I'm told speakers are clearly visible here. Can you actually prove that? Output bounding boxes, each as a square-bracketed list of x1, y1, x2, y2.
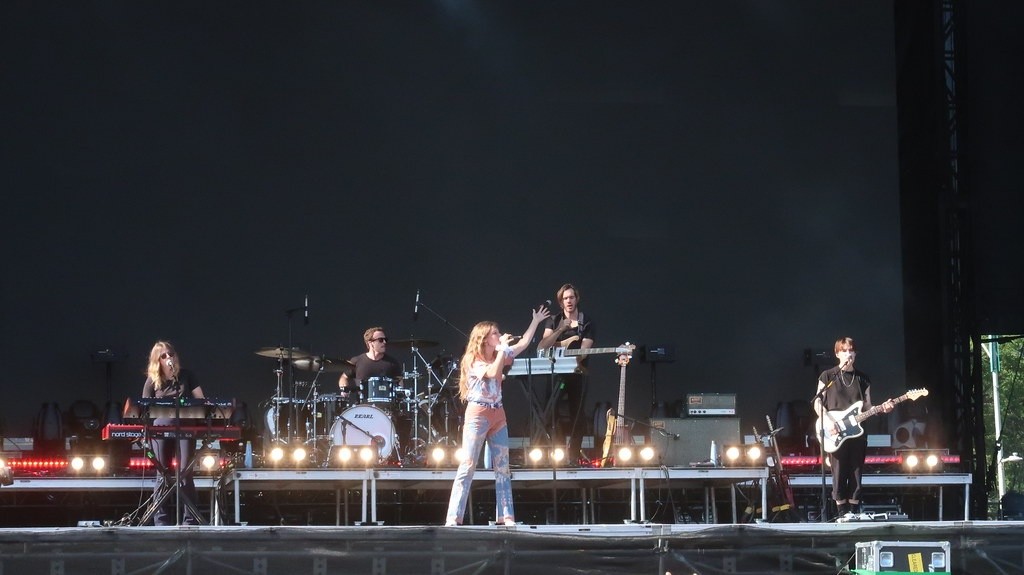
[649, 417, 741, 467]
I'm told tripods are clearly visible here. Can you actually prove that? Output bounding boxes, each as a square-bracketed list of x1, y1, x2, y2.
[296, 363, 330, 467]
[401, 346, 465, 468]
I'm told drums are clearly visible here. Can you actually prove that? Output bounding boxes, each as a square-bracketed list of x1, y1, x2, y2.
[272, 399, 326, 441]
[316, 392, 351, 440]
[359, 376, 394, 409]
[329, 404, 397, 464]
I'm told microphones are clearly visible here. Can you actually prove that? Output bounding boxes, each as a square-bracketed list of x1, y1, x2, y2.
[377, 439, 383, 445]
[304, 295, 309, 324]
[673, 434, 679, 440]
[847, 355, 853, 362]
[413, 290, 419, 321]
[166, 359, 174, 366]
[499, 336, 524, 342]
[541, 300, 552, 311]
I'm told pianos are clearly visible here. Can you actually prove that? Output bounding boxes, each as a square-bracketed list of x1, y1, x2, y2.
[101, 415, 244, 528]
[507, 354, 587, 447]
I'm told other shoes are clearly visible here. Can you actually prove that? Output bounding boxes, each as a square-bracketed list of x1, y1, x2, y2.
[444, 520, 458, 526]
[568, 460, 581, 468]
[844, 511, 871, 521]
[501, 521, 516, 525]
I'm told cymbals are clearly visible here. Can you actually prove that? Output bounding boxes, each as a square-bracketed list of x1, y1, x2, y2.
[290, 355, 357, 373]
[253, 346, 312, 359]
[389, 338, 440, 348]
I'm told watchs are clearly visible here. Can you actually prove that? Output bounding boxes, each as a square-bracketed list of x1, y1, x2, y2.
[340, 387, 349, 392]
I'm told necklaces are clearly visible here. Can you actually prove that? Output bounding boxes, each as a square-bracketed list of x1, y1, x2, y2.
[841, 368, 855, 387]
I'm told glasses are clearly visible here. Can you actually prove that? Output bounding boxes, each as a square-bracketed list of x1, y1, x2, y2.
[370, 337, 388, 342]
[160, 353, 174, 359]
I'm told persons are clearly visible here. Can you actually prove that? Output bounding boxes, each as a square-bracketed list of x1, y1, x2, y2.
[445, 305, 550, 526]
[338, 327, 415, 459]
[537, 284, 595, 467]
[813, 338, 894, 518]
[142, 341, 206, 526]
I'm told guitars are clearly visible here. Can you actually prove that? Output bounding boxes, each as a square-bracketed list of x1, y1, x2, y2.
[751, 414, 795, 521]
[601, 341, 635, 466]
[814, 386, 929, 455]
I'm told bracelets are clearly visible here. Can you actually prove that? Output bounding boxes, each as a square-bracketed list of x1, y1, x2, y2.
[496, 345, 511, 353]
